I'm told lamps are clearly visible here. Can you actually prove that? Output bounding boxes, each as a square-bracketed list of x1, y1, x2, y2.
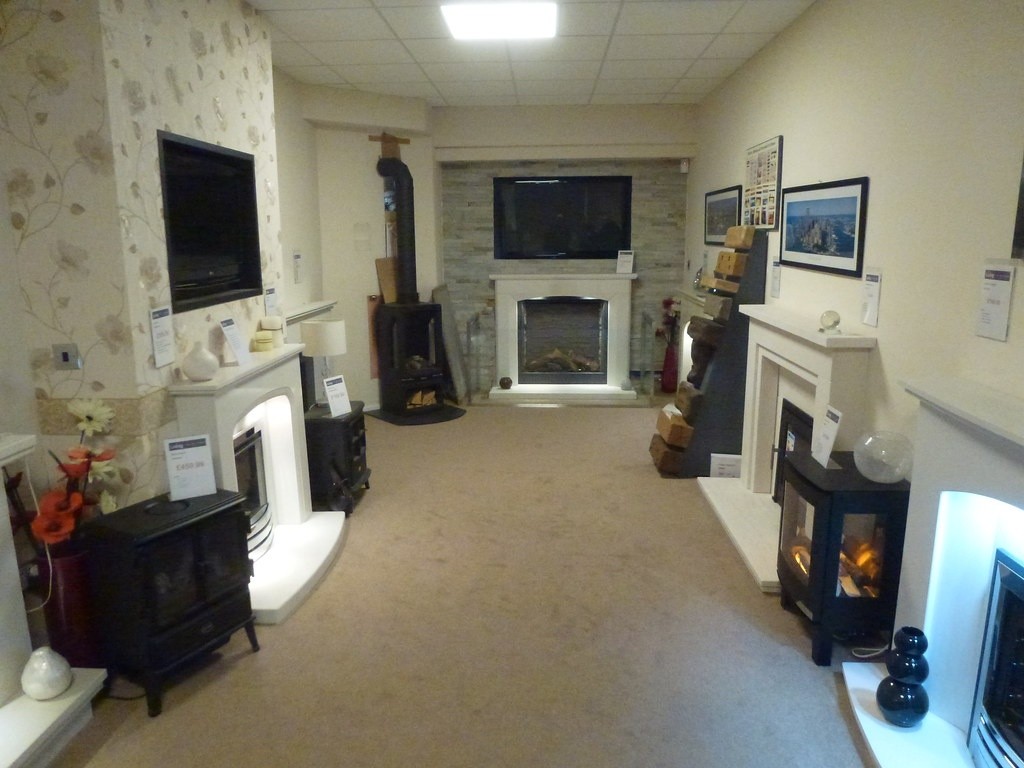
[300, 318, 346, 408]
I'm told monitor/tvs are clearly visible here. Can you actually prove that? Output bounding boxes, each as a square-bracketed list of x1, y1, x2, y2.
[163, 141, 250, 300]
[492, 175, 632, 260]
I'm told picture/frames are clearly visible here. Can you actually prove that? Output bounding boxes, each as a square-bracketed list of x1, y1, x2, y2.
[704, 185, 743, 246]
[779, 176, 869, 278]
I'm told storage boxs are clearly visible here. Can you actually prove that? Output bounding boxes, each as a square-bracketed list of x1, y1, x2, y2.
[649, 225, 756, 473]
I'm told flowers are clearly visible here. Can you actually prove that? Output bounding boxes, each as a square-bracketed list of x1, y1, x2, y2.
[28, 396, 119, 557]
[655, 298, 681, 346]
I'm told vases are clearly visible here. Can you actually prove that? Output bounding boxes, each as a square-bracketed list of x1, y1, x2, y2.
[21, 645, 73, 702]
[182, 341, 219, 382]
[876, 627, 930, 727]
[662, 346, 677, 393]
[38, 542, 116, 698]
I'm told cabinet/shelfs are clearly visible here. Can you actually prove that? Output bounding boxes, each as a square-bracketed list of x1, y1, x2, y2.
[674, 228, 768, 477]
[82, 488, 260, 717]
[304, 401, 372, 518]
